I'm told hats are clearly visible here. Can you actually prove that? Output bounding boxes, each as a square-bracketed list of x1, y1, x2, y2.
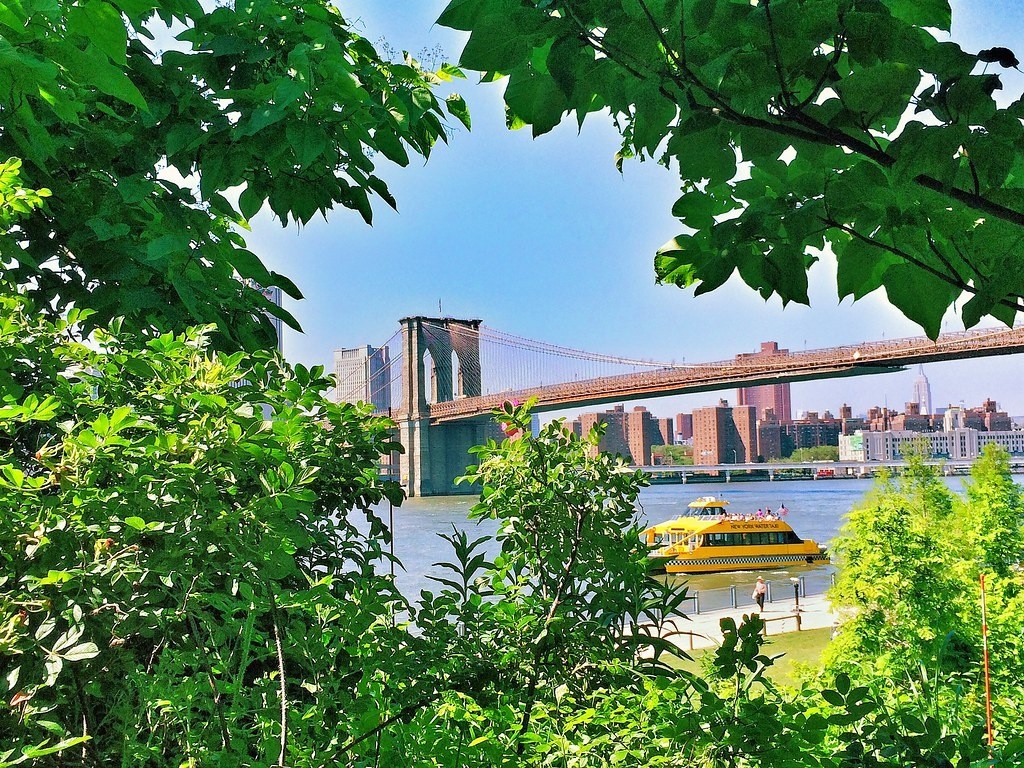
[756, 576, 764, 581]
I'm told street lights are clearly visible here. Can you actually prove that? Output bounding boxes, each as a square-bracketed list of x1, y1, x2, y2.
[733, 450, 737, 464]
[669, 451, 673, 465]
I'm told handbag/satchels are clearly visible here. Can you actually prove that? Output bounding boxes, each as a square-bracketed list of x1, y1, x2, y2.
[752, 589, 759, 600]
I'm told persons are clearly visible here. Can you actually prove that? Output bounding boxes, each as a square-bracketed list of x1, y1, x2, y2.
[717, 512, 745, 521]
[755, 575, 766, 612]
[749, 502, 790, 522]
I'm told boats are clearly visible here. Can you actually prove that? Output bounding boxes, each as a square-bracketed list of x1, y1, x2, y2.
[629, 495, 831, 574]
